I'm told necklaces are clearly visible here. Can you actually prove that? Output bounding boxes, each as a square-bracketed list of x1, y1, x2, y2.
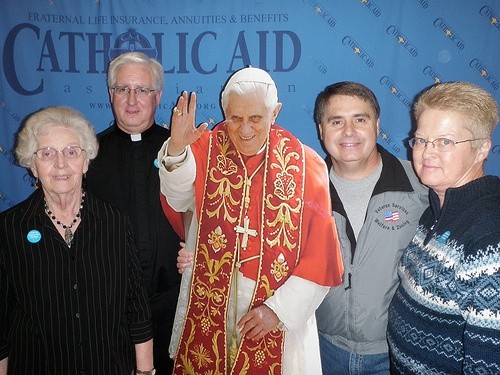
[44, 188, 86, 245]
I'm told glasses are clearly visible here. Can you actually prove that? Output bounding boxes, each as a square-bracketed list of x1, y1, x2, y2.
[112, 86, 155, 96]
[35, 146, 85, 161]
[408, 137, 487, 152]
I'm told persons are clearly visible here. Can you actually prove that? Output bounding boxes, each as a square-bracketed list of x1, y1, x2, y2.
[178, 81, 430, 375]
[386, 81, 500, 375]
[82, 50, 185, 374]
[0, 106, 156, 375]
[155, 66, 345, 374]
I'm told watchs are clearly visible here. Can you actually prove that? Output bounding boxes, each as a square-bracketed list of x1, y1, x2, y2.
[136, 368, 155, 374]
